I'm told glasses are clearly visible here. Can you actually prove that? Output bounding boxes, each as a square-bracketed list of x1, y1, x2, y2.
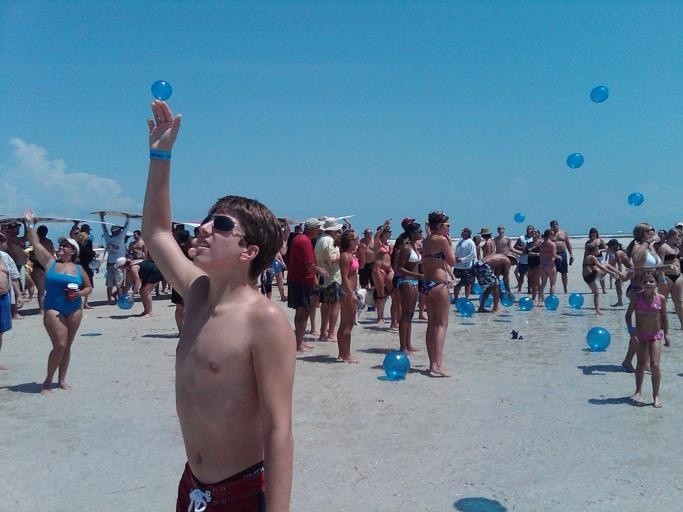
[383, 230, 392, 233]
[60, 244, 67, 247]
[440, 223, 451, 227]
[646, 227, 656, 232]
[415, 230, 424, 233]
[200, 214, 250, 246]
[498, 228, 505, 229]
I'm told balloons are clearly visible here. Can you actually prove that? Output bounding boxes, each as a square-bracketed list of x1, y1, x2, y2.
[586, 328, 611, 351]
[518, 297, 532, 311]
[628, 194, 643, 206]
[479, 294, 494, 308]
[569, 294, 583, 309]
[460, 301, 474, 318]
[456, 297, 467, 314]
[566, 154, 583, 169]
[500, 293, 515, 307]
[545, 295, 559, 311]
[514, 212, 524, 222]
[117, 294, 133, 310]
[473, 284, 483, 295]
[383, 352, 409, 380]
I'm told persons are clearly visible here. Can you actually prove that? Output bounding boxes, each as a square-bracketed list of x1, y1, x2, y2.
[31, 225, 55, 315]
[374, 218, 396, 329]
[172, 230, 193, 336]
[392, 217, 416, 257]
[68, 221, 80, 244]
[396, 223, 428, 354]
[515, 225, 536, 295]
[172, 224, 198, 245]
[629, 270, 671, 408]
[23, 243, 34, 301]
[154, 277, 172, 298]
[422, 213, 456, 376]
[550, 221, 574, 294]
[675, 222, 682, 277]
[112, 257, 163, 318]
[356, 228, 374, 291]
[79, 224, 95, 308]
[473, 235, 482, 261]
[605, 244, 619, 290]
[608, 240, 636, 307]
[583, 243, 624, 314]
[23, 207, 93, 395]
[626, 264, 641, 332]
[657, 228, 683, 330]
[0, 231, 23, 371]
[313, 218, 341, 341]
[476, 253, 517, 313]
[334, 224, 361, 255]
[141, 99, 295, 512]
[337, 230, 365, 363]
[127, 230, 147, 260]
[101, 212, 131, 305]
[493, 225, 523, 255]
[265, 253, 286, 301]
[287, 217, 330, 354]
[478, 228, 495, 257]
[452, 227, 477, 305]
[654, 230, 668, 250]
[622, 223, 662, 371]
[536, 230, 563, 307]
[513, 265, 528, 289]
[6, 221, 26, 319]
[416, 237, 427, 320]
[586, 228, 609, 295]
[527, 229, 543, 303]
[277, 218, 288, 278]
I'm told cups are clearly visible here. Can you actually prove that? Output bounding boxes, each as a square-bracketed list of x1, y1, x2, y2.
[66, 282, 78, 304]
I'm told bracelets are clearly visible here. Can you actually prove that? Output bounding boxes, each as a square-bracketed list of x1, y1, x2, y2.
[148, 151, 171, 161]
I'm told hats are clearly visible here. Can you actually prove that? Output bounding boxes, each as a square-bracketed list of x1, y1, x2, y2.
[113, 256, 126, 269]
[11, 223, 22, 228]
[305, 218, 325, 229]
[58, 235, 80, 257]
[111, 225, 121, 232]
[477, 227, 492, 235]
[319, 217, 343, 231]
[81, 224, 92, 231]
[36, 225, 48, 234]
[400, 217, 416, 229]
[675, 222, 683, 228]
